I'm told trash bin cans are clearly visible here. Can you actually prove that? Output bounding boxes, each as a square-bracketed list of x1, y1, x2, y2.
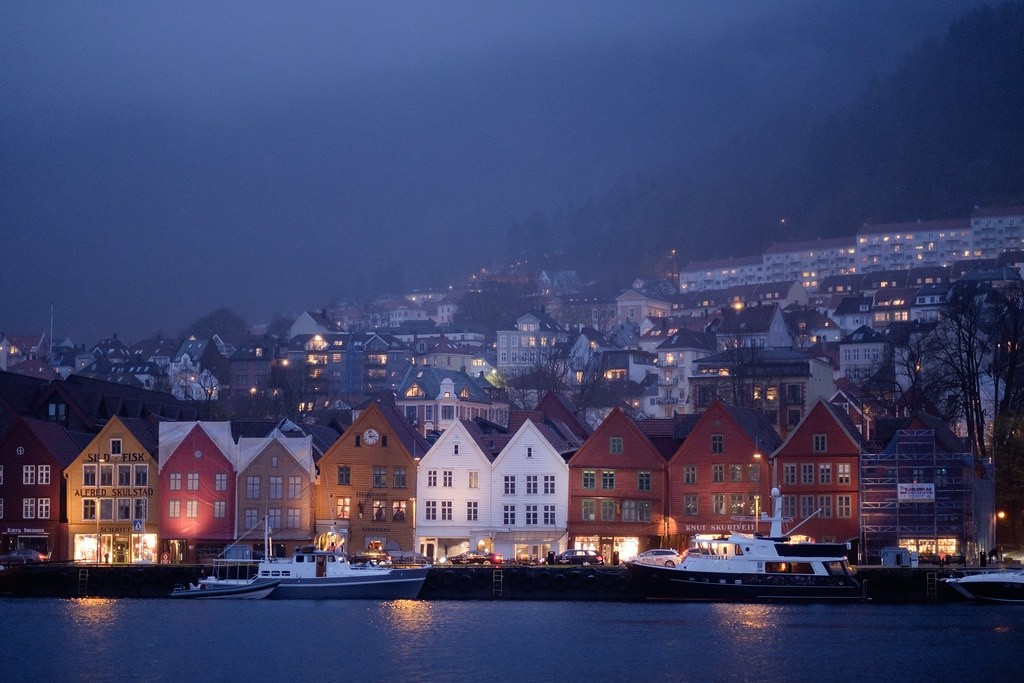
[979, 552, 986, 567]
[548, 550, 555, 565]
[613, 551, 619, 565]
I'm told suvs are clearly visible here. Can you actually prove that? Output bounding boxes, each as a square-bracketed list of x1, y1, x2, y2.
[632, 549, 683, 568]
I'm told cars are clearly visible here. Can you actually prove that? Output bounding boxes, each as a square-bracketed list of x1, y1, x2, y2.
[444, 549, 499, 566]
[0, 548, 44, 564]
[554, 549, 603, 566]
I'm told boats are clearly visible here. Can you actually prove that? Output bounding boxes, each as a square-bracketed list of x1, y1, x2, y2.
[168, 558, 282, 599]
[937, 567, 1024, 603]
[621, 509, 873, 604]
[257, 515, 433, 600]
